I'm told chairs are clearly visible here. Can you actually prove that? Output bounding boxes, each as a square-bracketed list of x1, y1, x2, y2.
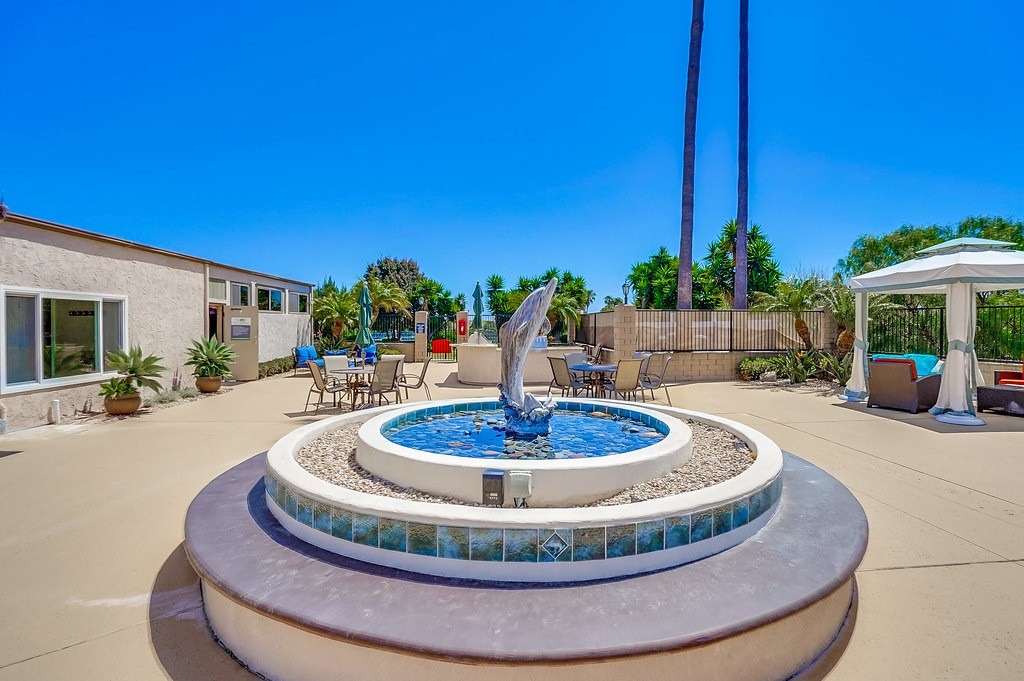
[547, 343, 673, 407]
[291, 343, 432, 415]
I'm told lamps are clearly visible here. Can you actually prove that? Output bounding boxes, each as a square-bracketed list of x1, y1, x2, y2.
[507, 470, 534, 508]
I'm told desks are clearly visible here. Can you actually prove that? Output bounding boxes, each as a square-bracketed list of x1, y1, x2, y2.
[330, 367, 376, 410]
[572, 364, 617, 398]
[347, 357, 367, 367]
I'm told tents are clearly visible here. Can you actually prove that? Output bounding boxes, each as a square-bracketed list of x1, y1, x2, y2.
[844, 238, 1024, 415]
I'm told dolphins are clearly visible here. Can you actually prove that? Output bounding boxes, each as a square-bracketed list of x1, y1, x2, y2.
[498, 277, 559, 414]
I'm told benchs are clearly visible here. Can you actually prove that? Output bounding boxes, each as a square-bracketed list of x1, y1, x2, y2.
[977, 371, 1024, 414]
[871, 353, 945, 377]
[865, 358, 942, 413]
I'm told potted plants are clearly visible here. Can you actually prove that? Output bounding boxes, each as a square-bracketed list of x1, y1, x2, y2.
[99, 344, 164, 414]
[185, 332, 240, 393]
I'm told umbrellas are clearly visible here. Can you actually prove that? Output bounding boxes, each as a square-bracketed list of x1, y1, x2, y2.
[355, 281, 375, 369]
[471, 281, 484, 346]
